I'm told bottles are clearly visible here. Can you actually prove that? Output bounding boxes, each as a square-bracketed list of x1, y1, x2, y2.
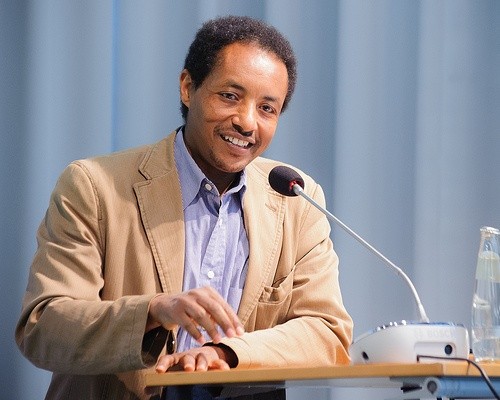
[471, 225, 499, 363]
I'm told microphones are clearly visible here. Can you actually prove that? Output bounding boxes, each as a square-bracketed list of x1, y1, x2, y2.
[269, 165, 469, 363]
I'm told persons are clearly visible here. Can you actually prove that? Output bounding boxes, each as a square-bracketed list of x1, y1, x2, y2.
[13, 13, 354, 400]
